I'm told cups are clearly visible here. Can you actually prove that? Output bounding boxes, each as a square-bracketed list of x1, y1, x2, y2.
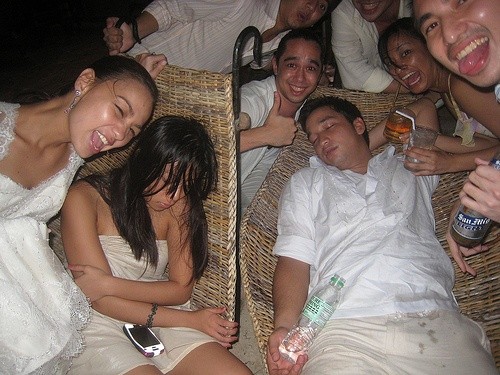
[384, 105, 415, 142]
[404, 125, 438, 172]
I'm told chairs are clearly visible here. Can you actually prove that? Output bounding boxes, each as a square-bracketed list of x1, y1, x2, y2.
[237, 85, 500, 372]
[52, 25, 262, 348]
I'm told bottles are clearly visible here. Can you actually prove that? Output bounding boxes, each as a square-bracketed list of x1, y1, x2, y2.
[450, 149, 500, 246]
[280, 274, 344, 362]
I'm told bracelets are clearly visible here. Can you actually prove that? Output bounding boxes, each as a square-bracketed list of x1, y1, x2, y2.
[131, 18, 142, 44]
[145, 303, 158, 329]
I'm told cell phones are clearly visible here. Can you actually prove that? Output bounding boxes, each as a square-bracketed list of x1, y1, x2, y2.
[124, 323, 166, 357]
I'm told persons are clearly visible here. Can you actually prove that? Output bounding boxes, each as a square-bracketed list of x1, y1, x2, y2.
[102, 0, 342, 74]
[266, 94, 500, 375]
[378, 0, 500, 224]
[60, 115, 254, 375]
[0, 55, 159, 375]
[331, 0, 415, 94]
[240, 29, 326, 153]
